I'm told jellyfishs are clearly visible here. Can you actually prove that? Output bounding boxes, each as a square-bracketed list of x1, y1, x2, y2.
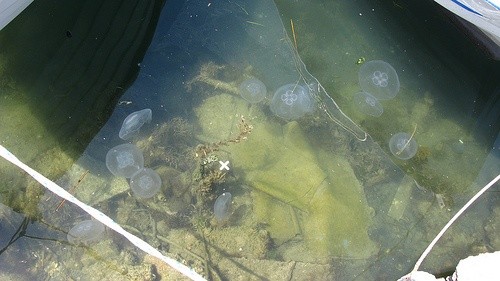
[351, 92, 385, 118]
[66, 218, 105, 246]
[358, 60, 399, 100]
[105, 143, 145, 179]
[239, 77, 267, 105]
[118, 109, 152, 141]
[130, 168, 161, 198]
[389, 131, 418, 160]
[269, 83, 313, 121]
[213, 191, 234, 226]
